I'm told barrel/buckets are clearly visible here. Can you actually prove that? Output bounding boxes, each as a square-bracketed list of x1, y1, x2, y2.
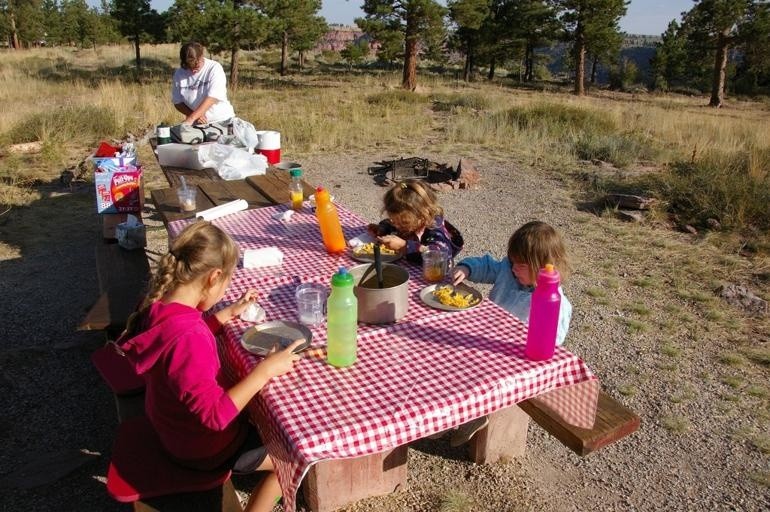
[254, 130, 281, 164]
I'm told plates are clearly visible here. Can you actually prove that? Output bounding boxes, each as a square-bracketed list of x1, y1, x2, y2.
[349, 232, 404, 263]
[420, 281, 483, 312]
[242, 320, 311, 355]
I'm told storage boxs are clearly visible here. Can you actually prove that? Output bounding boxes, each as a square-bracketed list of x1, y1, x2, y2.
[94, 167, 144, 215]
[91, 148, 137, 172]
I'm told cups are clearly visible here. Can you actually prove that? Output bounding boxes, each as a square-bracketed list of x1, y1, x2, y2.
[420, 249, 450, 282]
[295, 285, 327, 327]
[179, 190, 198, 212]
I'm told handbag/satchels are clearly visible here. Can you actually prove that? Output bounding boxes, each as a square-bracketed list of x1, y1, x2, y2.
[170, 122, 237, 144]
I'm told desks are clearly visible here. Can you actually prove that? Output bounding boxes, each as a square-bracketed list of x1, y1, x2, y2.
[149, 138, 284, 188]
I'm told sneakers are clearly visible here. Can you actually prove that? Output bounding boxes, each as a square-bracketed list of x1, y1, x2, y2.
[451, 415, 490, 449]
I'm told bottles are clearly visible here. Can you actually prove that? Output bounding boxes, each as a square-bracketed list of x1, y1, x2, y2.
[315, 186, 347, 253]
[289, 170, 304, 211]
[328, 268, 361, 370]
[157, 122, 171, 145]
[524, 264, 561, 362]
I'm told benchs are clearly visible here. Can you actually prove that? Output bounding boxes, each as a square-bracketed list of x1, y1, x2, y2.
[518, 378, 640, 458]
[94, 243, 245, 512]
[102, 214, 146, 241]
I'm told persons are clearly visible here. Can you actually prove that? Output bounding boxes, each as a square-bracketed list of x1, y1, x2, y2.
[171, 42, 236, 126]
[427, 220, 573, 448]
[366, 179, 464, 269]
[113, 220, 307, 512]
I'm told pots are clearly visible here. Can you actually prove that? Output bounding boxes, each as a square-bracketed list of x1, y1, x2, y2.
[346, 263, 410, 322]
